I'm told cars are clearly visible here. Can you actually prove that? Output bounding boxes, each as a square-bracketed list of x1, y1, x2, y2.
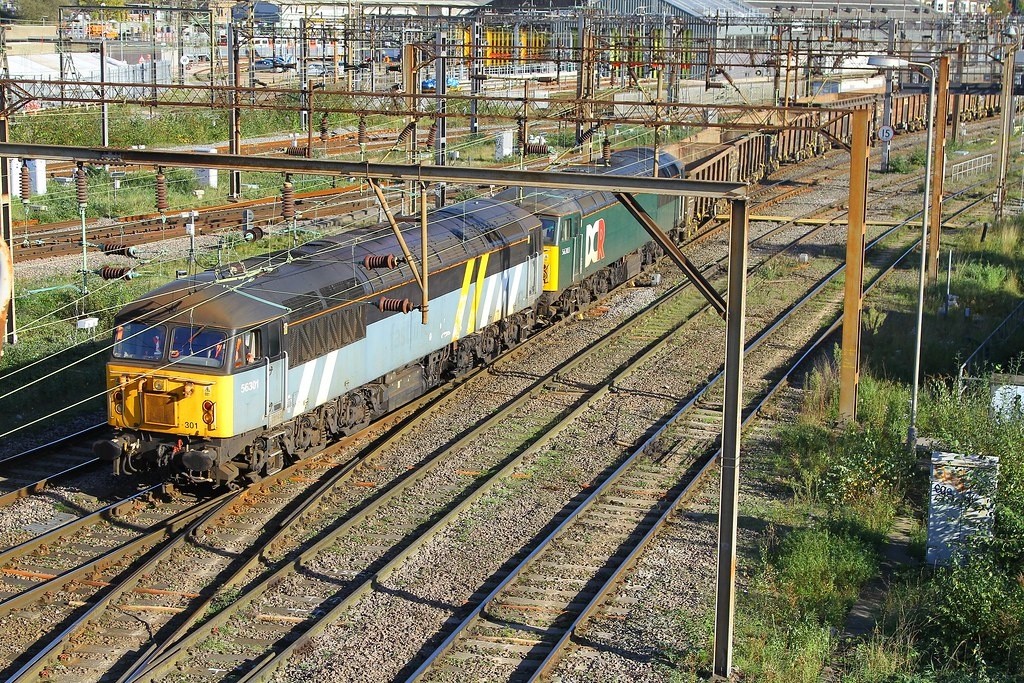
[250, 56, 371, 77]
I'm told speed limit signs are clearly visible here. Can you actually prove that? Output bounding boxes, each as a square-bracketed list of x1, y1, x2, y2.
[877, 125, 894, 142]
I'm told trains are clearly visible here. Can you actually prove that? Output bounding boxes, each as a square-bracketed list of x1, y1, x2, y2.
[93, 81, 1024, 494]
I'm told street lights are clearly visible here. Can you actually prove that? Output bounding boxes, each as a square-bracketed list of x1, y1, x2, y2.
[100, 2, 106, 37]
[867, 57, 936, 467]
[995, 26, 1022, 221]
[109, 20, 123, 61]
[401, 27, 424, 102]
